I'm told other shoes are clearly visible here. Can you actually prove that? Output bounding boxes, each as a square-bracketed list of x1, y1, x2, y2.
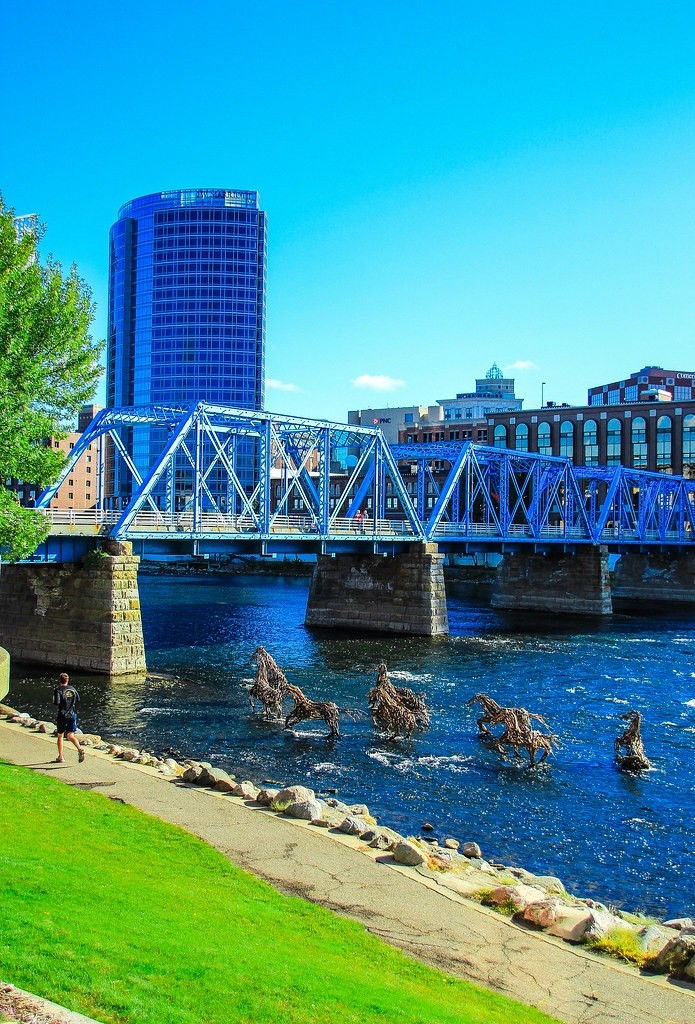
[56, 757, 65, 762]
[78, 748, 85, 763]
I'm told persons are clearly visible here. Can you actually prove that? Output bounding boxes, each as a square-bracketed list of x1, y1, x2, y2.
[26, 496, 36, 508]
[53, 673, 85, 763]
[354, 509, 368, 534]
